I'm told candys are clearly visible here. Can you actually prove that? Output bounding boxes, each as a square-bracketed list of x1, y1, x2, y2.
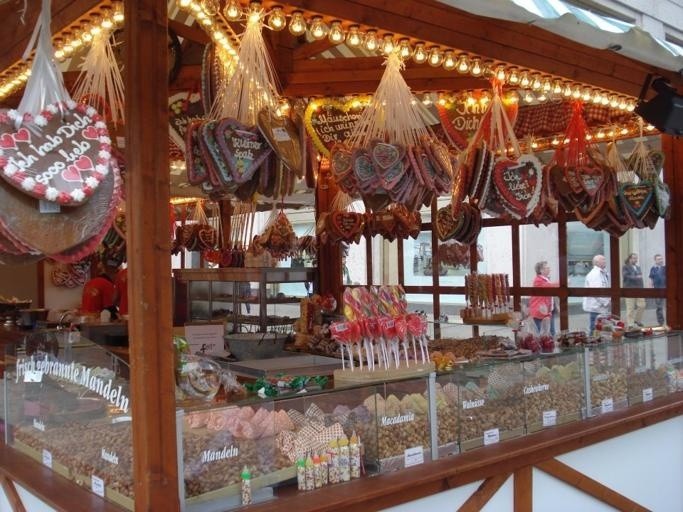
[465, 272, 510, 320]
[321, 284, 431, 371]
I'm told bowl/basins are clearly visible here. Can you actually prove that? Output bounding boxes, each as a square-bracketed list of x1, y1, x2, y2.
[224, 333, 289, 360]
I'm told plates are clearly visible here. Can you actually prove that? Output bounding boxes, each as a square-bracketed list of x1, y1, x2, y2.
[540, 351, 560, 358]
[454, 358, 469, 364]
[474, 349, 532, 360]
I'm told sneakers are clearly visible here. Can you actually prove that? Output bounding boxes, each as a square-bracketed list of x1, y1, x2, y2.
[634, 320, 644, 326]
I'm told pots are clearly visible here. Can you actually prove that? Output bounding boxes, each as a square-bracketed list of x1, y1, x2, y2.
[17, 309, 48, 328]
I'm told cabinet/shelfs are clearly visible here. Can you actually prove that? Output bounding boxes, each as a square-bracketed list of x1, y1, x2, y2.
[172, 268, 318, 333]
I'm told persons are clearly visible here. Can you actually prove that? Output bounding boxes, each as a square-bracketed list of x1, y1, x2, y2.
[528, 261, 559, 336]
[621, 253, 646, 329]
[78, 272, 118, 320]
[582, 254, 612, 336]
[648, 254, 667, 327]
[113, 268, 127, 315]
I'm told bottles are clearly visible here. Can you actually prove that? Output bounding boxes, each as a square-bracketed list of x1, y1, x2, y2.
[241, 465, 253, 507]
[298, 431, 362, 492]
